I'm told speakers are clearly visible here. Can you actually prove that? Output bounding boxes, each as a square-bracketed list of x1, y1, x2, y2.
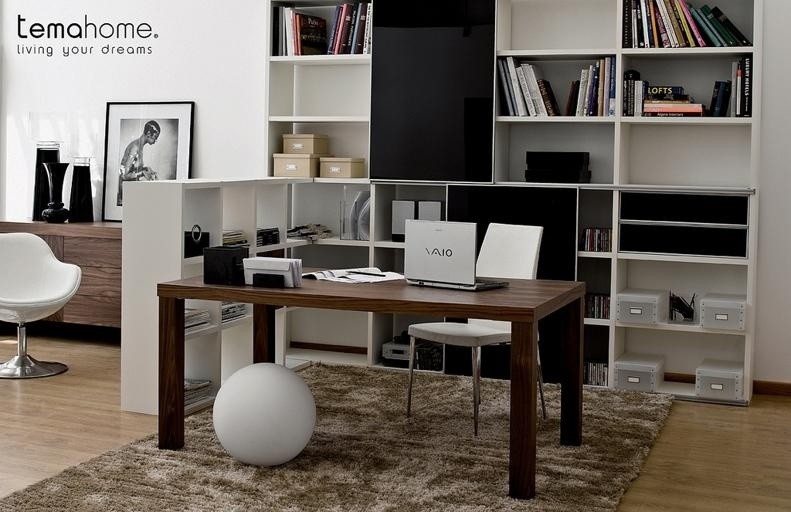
[391, 199, 416, 241]
[418, 199, 442, 221]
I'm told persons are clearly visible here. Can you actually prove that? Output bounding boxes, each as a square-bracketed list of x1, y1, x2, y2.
[116, 118, 162, 207]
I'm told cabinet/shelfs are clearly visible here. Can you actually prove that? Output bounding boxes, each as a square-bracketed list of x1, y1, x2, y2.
[3, 216, 120, 338]
[490, 0, 763, 185]
[289, 181, 761, 409]
[123, 171, 311, 422]
[263, 1, 378, 179]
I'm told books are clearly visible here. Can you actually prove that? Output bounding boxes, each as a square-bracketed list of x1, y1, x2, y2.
[273, 1, 374, 56]
[496, 57, 616, 117]
[582, 224, 613, 387]
[622, 0, 753, 118]
[302, 265, 408, 284]
[184, 298, 249, 408]
[222, 222, 282, 249]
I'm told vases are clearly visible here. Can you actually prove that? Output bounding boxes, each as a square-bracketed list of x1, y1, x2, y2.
[41, 162, 69, 224]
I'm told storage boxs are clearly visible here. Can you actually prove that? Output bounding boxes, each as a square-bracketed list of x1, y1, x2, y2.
[278, 131, 328, 156]
[317, 157, 366, 179]
[271, 153, 320, 179]
[692, 361, 740, 398]
[701, 291, 746, 332]
[611, 351, 665, 393]
[616, 285, 669, 324]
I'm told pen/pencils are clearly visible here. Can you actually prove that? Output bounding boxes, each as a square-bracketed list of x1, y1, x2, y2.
[348, 271, 385, 277]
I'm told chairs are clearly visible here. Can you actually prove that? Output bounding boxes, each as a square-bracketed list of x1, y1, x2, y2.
[0, 231, 78, 381]
[405, 220, 549, 440]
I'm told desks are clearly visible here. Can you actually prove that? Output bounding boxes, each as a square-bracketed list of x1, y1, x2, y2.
[151, 267, 585, 503]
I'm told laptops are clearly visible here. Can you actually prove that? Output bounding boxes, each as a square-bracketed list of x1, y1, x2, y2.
[401, 219, 509, 292]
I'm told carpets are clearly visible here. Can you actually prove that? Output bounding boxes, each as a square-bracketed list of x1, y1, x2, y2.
[1, 355, 671, 512]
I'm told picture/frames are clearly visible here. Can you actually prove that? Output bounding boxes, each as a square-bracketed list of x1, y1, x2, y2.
[102, 96, 194, 224]
